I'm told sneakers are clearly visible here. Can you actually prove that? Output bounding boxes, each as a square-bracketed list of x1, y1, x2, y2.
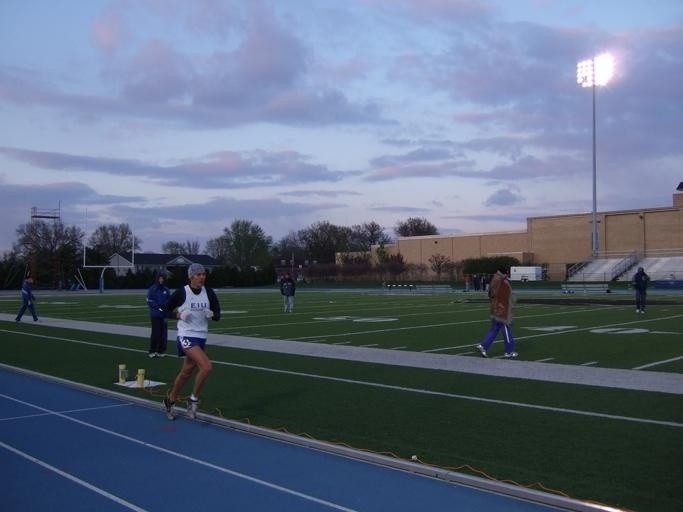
[187, 396, 201, 419]
[504, 352, 520, 359]
[148, 353, 156, 358]
[476, 345, 490, 360]
[158, 352, 169, 358]
[163, 391, 178, 420]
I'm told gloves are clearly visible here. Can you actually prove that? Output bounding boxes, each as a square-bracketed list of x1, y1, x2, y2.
[179, 308, 193, 320]
[204, 307, 214, 319]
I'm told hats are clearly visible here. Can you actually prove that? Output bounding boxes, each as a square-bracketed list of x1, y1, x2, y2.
[187, 262, 207, 278]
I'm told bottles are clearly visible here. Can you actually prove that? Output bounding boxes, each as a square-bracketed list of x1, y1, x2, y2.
[136, 369, 146, 388]
[119, 364, 127, 384]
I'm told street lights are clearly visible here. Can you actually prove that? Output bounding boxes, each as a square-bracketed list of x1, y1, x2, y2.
[576, 52, 617, 255]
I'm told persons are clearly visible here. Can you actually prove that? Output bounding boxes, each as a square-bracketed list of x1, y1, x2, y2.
[144, 273, 169, 358]
[631, 266, 650, 313]
[463, 272, 487, 290]
[162, 263, 221, 422]
[14, 275, 38, 322]
[474, 266, 518, 358]
[279, 271, 294, 312]
